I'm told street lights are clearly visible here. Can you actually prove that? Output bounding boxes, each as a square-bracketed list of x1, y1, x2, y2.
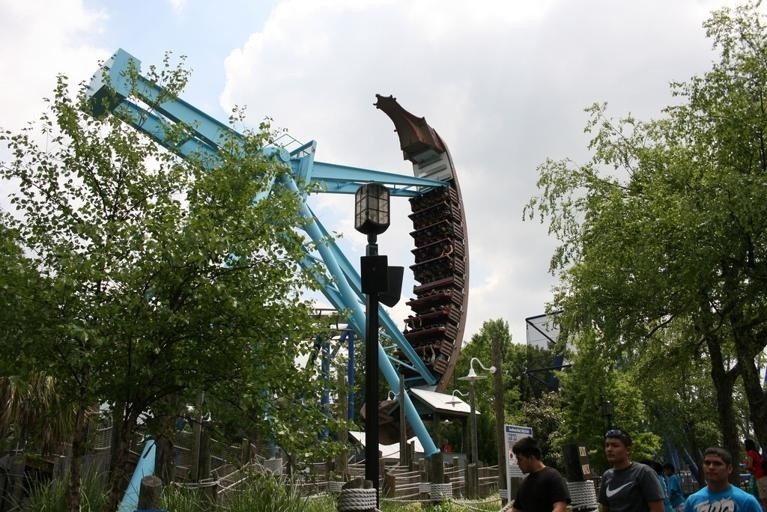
[355, 184, 390, 507]
[601, 396, 614, 426]
[448, 340, 508, 508]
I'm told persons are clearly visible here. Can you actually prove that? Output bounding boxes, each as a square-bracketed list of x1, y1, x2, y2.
[175, 411, 189, 432]
[440, 437, 452, 453]
[506, 436, 571, 512]
[597, 428, 767, 512]
[397, 188, 457, 368]
